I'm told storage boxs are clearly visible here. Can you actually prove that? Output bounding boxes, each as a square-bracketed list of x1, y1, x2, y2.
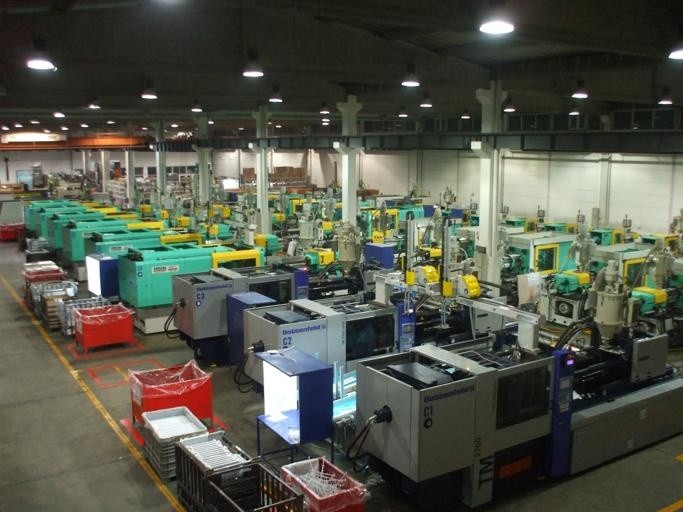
[21, 260, 138, 353]
[127, 359, 365, 511]
